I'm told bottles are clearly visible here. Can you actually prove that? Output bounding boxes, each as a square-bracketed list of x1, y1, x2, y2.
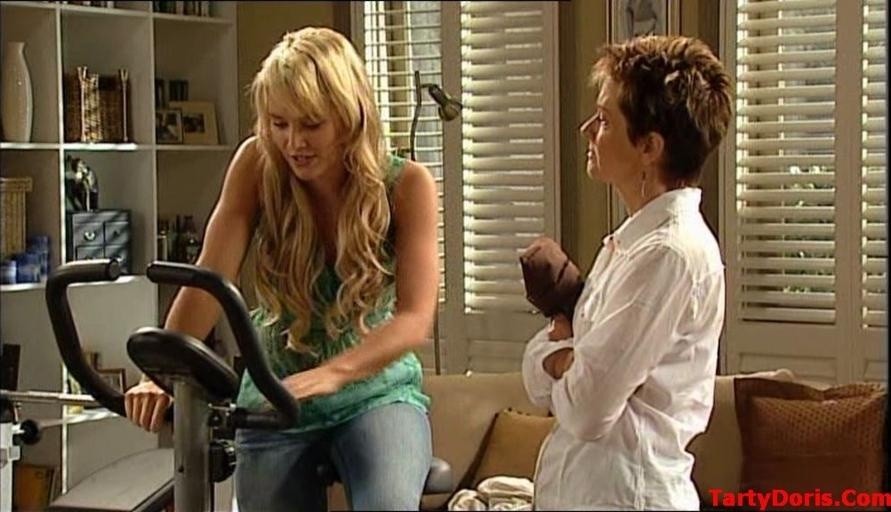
[172, 215, 203, 264]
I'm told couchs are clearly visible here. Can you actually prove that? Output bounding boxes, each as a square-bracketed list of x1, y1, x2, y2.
[416, 360, 887, 510]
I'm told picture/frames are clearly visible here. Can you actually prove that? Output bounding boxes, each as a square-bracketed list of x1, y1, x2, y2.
[157, 99, 223, 147]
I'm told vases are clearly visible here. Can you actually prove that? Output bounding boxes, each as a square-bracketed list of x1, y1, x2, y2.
[2, 39, 37, 142]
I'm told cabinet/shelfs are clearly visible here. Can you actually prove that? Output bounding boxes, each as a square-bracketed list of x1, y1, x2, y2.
[0, 2, 241, 506]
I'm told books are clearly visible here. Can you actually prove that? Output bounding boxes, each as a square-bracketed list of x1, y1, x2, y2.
[29, 0, 216, 17]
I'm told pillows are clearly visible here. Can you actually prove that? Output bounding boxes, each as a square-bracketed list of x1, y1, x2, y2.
[426, 369, 553, 489]
[689, 365, 889, 510]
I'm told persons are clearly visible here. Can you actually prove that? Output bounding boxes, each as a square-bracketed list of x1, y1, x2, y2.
[520, 35, 732, 511]
[126, 26, 439, 512]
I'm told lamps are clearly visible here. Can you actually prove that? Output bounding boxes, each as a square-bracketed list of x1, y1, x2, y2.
[408, 68, 466, 377]
